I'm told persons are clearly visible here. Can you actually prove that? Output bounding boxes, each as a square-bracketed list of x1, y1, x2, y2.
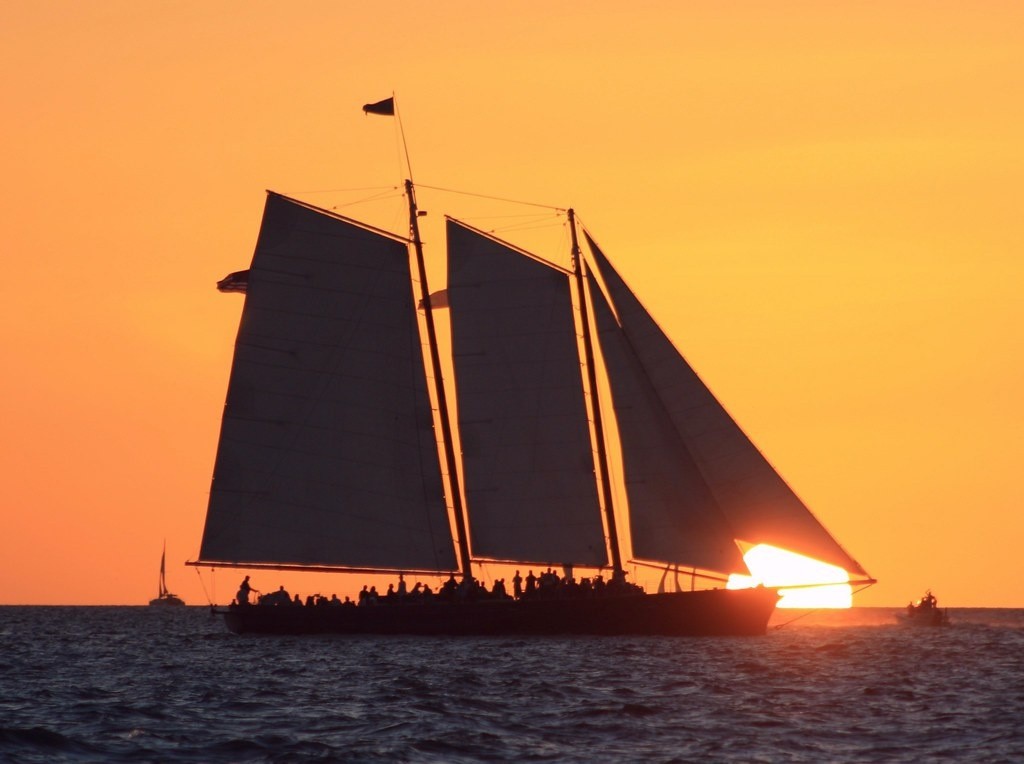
[907, 589, 936, 617]
[236, 576, 259, 606]
[278, 567, 613, 607]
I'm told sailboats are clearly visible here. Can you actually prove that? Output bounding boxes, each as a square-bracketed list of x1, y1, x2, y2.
[181, 88, 878, 637]
[148, 538, 187, 606]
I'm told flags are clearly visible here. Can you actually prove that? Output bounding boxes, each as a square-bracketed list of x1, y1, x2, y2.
[362, 97, 394, 116]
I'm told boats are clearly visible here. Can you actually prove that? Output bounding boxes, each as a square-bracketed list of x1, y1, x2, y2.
[894, 590, 953, 628]
